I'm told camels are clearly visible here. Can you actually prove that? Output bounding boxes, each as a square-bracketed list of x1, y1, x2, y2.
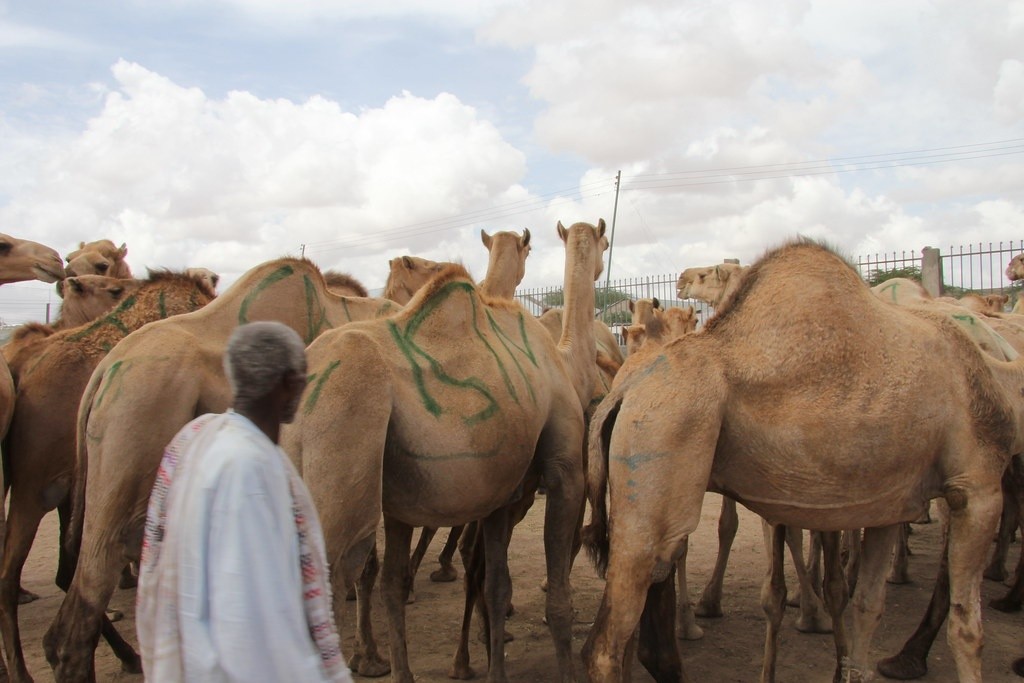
[0, 215, 1023, 682]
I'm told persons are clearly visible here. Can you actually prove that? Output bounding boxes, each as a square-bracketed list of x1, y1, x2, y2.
[136, 321, 355, 683]
